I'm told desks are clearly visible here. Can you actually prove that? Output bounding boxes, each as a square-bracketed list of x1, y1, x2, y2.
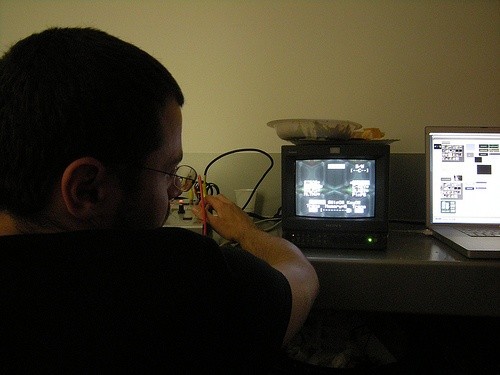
[161, 217, 500, 317]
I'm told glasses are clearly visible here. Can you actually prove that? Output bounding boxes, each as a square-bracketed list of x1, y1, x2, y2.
[56, 162, 197, 192]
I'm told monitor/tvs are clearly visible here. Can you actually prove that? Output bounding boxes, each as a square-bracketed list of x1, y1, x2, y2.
[281, 144, 389, 252]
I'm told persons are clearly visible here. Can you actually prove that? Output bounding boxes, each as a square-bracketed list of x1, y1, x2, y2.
[0, 27, 318, 375]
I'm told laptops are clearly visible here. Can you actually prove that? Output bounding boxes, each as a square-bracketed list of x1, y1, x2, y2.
[424, 126, 500, 259]
